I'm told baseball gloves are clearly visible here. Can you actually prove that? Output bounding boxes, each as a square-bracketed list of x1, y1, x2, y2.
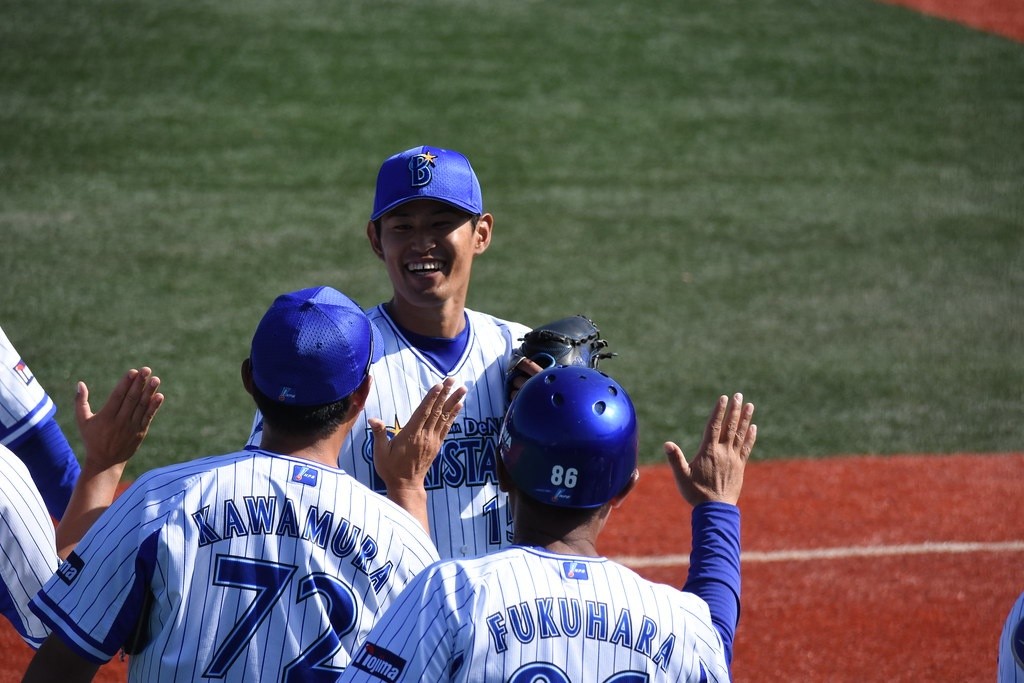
[504, 316, 618, 414]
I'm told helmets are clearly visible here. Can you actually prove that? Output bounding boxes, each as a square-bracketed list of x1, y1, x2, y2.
[499, 365, 638, 511]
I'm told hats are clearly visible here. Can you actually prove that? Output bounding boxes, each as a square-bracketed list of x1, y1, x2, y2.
[370, 144, 483, 224]
[250, 285, 385, 408]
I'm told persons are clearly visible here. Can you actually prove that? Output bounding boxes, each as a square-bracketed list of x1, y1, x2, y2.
[247, 146, 547, 561]
[18, 285, 443, 683]
[0, 321, 166, 648]
[337, 364, 759, 683]
